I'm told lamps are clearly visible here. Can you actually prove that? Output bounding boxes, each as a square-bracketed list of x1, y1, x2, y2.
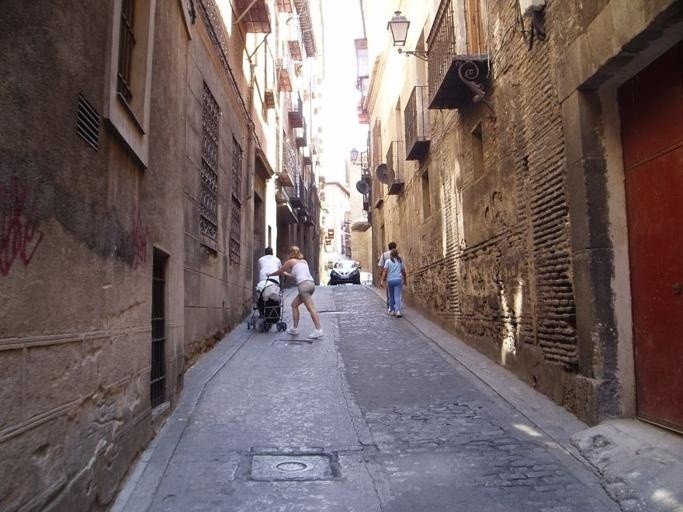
[350, 149, 369, 167]
[387, 11, 428, 60]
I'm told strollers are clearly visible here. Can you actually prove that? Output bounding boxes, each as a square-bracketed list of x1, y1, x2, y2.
[247, 274, 287, 332]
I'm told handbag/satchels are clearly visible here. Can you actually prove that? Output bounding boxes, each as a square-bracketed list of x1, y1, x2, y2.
[379, 266, 386, 278]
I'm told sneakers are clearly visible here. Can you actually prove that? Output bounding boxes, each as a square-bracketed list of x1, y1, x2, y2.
[308, 328, 322, 338]
[387, 306, 402, 317]
[287, 327, 297, 334]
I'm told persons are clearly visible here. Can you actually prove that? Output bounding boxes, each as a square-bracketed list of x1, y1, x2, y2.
[377, 242, 402, 309]
[379, 250, 414, 318]
[256, 246, 284, 288]
[267, 247, 326, 339]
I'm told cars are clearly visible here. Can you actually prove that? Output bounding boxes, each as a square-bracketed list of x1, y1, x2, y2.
[330, 260, 361, 284]
